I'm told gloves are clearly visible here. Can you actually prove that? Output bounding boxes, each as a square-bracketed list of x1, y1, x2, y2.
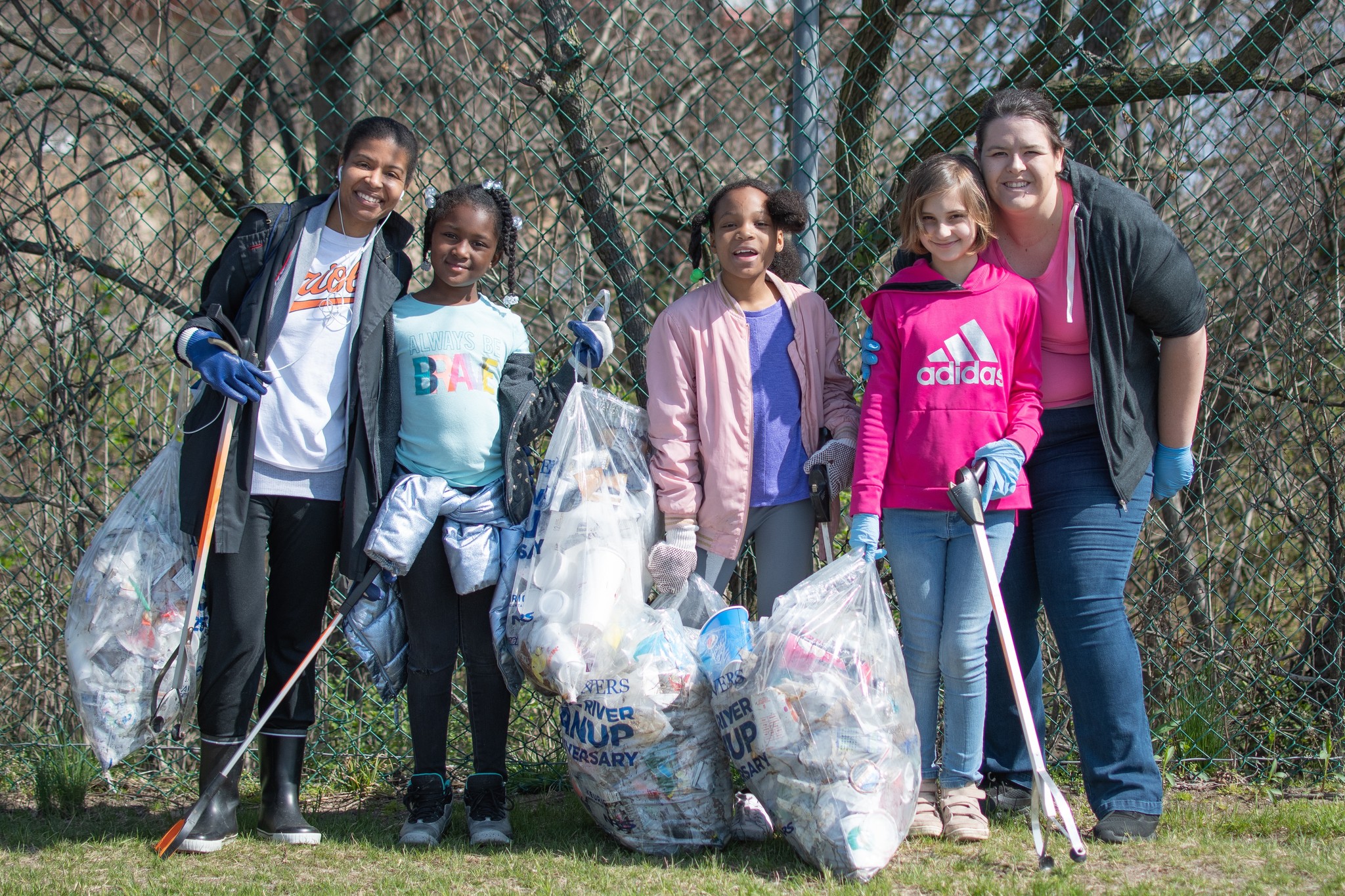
[647, 524, 700, 593]
[803, 438, 858, 502]
[177, 329, 273, 404]
[1151, 440, 1194, 501]
[848, 511, 887, 562]
[971, 438, 1025, 512]
[568, 305, 616, 377]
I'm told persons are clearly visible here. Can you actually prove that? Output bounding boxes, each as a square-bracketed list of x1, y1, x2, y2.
[851, 151, 1043, 844]
[381, 184, 614, 852]
[647, 178, 858, 843]
[174, 116, 420, 854]
[859, 88, 1210, 843]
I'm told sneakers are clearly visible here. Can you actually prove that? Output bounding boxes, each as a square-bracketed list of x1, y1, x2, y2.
[939, 780, 989, 841]
[398, 773, 454, 845]
[731, 791, 774, 840]
[463, 772, 516, 847]
[908, 778, 944, 838]
[976, 771, 1032, 823]
[1094, 810, 1159, 842]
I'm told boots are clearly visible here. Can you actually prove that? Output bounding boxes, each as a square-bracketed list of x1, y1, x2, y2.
[178, 733, 246, 854]
[256, 728, 322, 844]
